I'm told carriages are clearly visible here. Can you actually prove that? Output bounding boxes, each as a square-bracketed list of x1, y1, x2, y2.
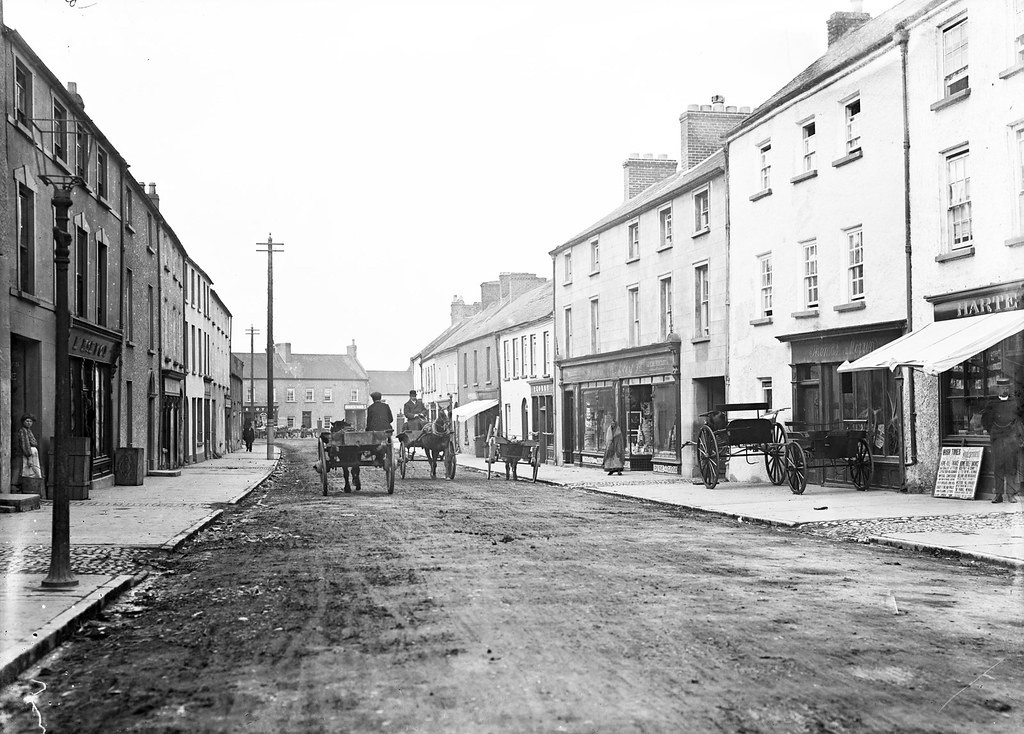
[312, 419, 397, 496]
[395, 401, 457, 481]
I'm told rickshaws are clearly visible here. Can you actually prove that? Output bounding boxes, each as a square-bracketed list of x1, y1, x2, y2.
[676, 408, 791, 489]
[783, 419, 875, 495]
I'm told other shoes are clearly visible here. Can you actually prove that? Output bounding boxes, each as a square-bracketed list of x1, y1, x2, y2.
[249, 449, 251, 452]
[618, 470, 622, 475]
[608, 471, 613, 475]
[246, 447, 249, 451]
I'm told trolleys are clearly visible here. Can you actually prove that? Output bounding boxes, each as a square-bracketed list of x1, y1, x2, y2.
[485, 434, 541, 482]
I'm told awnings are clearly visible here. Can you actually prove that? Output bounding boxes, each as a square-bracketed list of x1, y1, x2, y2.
[452, 399, 499, 423]
[837, 309, 1024, 374]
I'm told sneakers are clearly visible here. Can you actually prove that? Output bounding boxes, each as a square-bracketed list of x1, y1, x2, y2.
[992, 494, 1003, 503]
[1008, 494, 1017, 503]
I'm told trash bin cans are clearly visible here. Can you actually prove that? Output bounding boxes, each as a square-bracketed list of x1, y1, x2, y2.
[47, 436, 92, 500]
[115, 447, 144, 487]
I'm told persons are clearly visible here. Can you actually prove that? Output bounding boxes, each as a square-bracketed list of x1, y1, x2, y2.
[981, 379, 1020, 503]
[602, 419, 625, 475]
[301, 422, 306, 428]
[404, 390, 428, 430]
[17, 411, 43, 497]
[365, 392, 393, 432]
[243, 423, 254, 452]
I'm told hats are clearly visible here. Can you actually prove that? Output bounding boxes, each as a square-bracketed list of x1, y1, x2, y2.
[370, 392, 382, 400]
[409, 390, 417, 395]
[248, 423, 252, 426]
[995, 377, 1013, 387]
[611, 421, 615, 424]
